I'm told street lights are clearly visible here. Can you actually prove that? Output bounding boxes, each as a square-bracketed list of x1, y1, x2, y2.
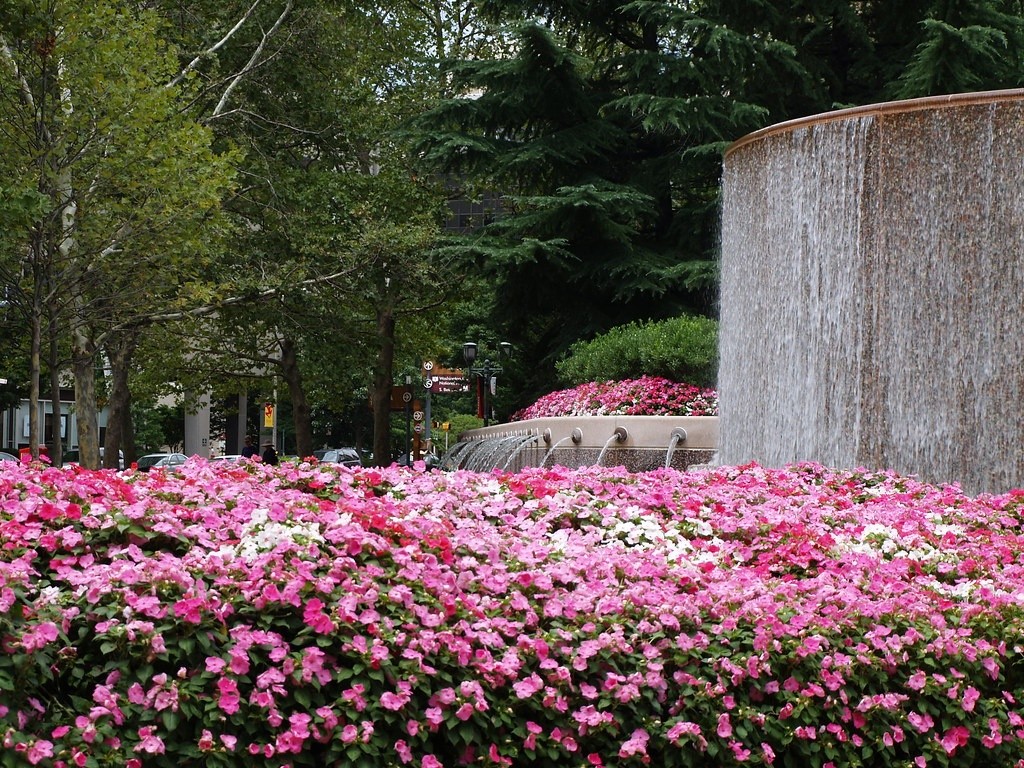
[405, 375, 411, 467]
[463, 342, 511, 428]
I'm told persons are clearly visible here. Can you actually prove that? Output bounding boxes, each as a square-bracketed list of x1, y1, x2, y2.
[261, 439, 278, 466]
[241, 435, 257, 458]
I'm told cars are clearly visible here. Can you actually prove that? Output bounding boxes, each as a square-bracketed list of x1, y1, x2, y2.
[321, 449, 361, 466]
[61, 447, 124, 466]
[396, 453, 439, 467]
[211, 456, 241, 463]
[132, 453, 188, 471]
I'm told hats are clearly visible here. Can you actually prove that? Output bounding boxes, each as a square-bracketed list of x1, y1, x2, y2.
[260, 439, 274, 447]
